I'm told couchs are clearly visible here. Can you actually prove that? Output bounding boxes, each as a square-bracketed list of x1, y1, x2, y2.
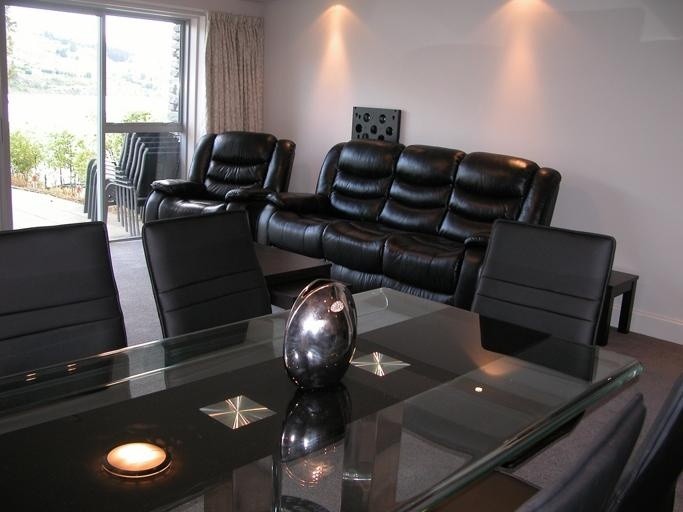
[143, 131, 297, 247]
[256, 141, 562, 310]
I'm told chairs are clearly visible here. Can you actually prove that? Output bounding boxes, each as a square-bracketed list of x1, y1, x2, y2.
[0, 221, 128, 381]
[143, 209, 275, 388]
[471, 219, 617, 346]
